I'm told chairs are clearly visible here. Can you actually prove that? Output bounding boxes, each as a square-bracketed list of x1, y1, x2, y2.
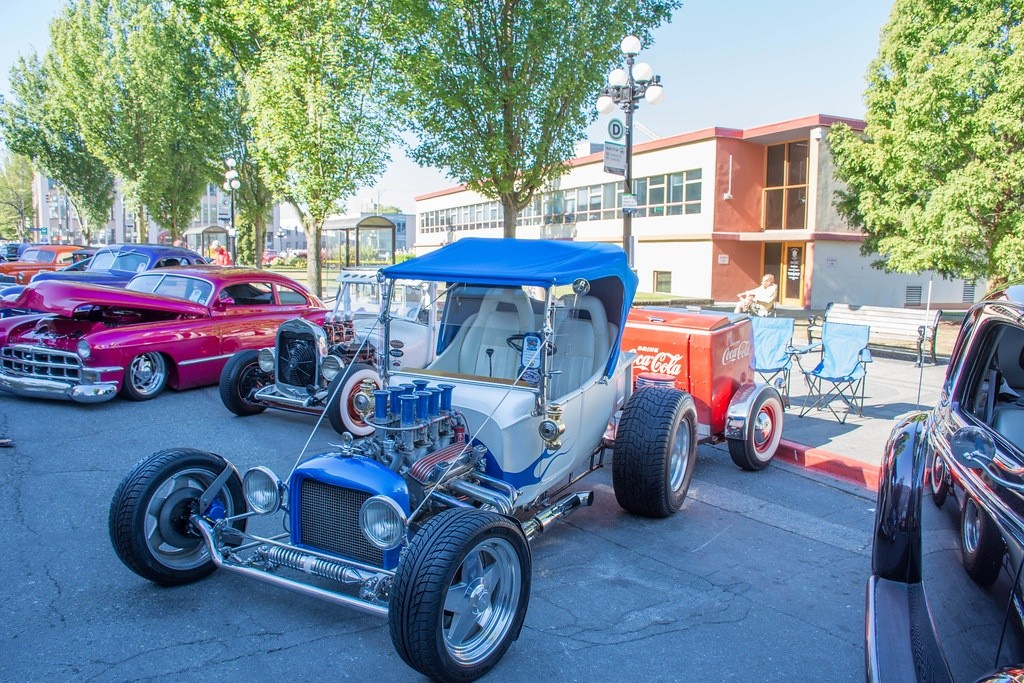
[997, 326, 1023, 450]
[540, 294, 609, 401]
[798, 322, 873, 425]
[744, 317, 795, 408]
[459, 288, 536, 379]
[395, 288, 424, 317]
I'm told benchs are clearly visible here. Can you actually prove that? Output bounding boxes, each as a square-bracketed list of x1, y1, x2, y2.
[806, 300, 943, 368]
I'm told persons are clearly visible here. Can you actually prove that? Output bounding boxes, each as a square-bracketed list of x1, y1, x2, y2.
[210, 240, 231, 265]
[734, 273, 778, 313]
[17, 238, 32, 257]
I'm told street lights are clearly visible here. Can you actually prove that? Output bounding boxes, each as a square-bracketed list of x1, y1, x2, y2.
[222, 158, 240, 263]
[595, 34, 666, 271]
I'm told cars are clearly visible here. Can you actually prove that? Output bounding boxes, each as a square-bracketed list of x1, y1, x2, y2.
[109, 238, 698, 683]
[218, 271, 440, 438]
[0, 242, 212, 295]
[865, 277, 1024, 683]
[0, 264, 349, 403]
